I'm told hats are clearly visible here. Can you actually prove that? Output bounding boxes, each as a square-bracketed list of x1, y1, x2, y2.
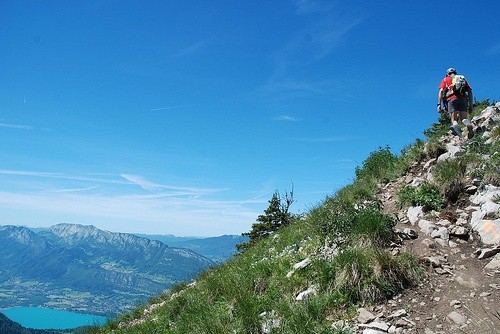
[446, 67, 457, 74]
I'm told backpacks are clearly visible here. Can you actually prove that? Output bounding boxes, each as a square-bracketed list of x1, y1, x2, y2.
[446, 75, 467, 95]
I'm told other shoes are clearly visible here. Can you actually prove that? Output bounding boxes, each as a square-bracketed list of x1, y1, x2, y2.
[451, 124, 460, 135]
[467, 124, 474, 138]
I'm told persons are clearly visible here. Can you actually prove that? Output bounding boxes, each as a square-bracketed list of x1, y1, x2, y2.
[437, 67, 475, 145]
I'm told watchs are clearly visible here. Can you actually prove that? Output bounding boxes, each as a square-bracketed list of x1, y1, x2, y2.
[437, 103, 441, 106]
